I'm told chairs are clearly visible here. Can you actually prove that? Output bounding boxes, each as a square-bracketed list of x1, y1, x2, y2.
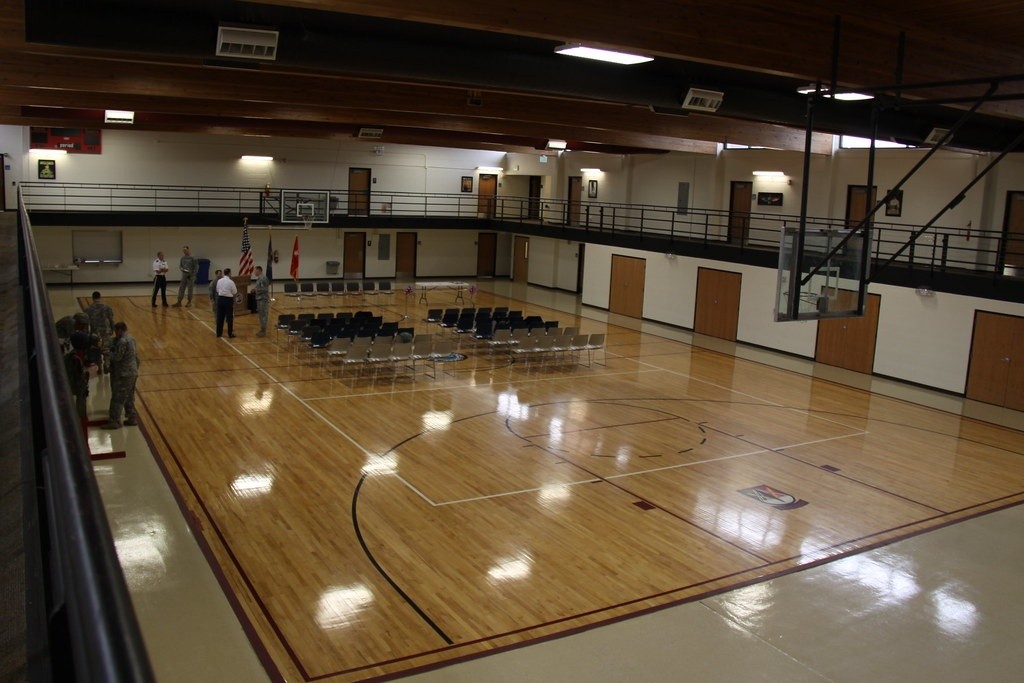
[377, 280, 394, 305]
[422, 307, 608, 375]
[273, 312, 456, 386]
[331, 283, 346, 309]
[317, 282, 332, 308]
[299, 283, 315, 307]
[282, 283, 301, 309]
[362, 281, 378, 307]
[347, 282, 363, 308]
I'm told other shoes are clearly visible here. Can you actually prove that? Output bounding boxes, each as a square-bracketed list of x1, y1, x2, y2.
[162, 303, 168, 306]
[229, 333, 236, 337]
[100, 419, 121, 429]
[171, 302, 182, 307]
[185, 303, 192, 307]
[97, 370, 102, 374]
[256, 330, 266, 336]
[152, 304, 158, 307]
[104, 368, 108, 374]
[123, 417, 137, 426]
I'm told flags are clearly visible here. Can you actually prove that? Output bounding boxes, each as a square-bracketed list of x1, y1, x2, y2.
[289, 234, 300, 282]
[238, 216, 254, 277]
[265, 230, 273, 284]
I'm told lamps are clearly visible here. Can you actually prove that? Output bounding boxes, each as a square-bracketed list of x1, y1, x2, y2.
[797, 82, 875, 102]
[555, 42, 654, 66]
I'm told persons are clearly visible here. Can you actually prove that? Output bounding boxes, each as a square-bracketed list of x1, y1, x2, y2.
[151, 251, 169, 307]
[250, 266, 270, 337]
[55, 290, 141, 430]
[208, 268, 238, 339]
[171, 245, 199, 308]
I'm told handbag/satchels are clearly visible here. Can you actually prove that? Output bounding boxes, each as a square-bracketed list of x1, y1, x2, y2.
[70, 355, 88, 395]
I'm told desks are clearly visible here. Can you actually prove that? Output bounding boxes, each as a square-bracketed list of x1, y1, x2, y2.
[415, 281, 470, 306]
[40, 265, 80, 288]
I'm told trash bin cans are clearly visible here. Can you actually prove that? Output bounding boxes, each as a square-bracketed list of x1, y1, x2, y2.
[194, 259, 211, 284]
